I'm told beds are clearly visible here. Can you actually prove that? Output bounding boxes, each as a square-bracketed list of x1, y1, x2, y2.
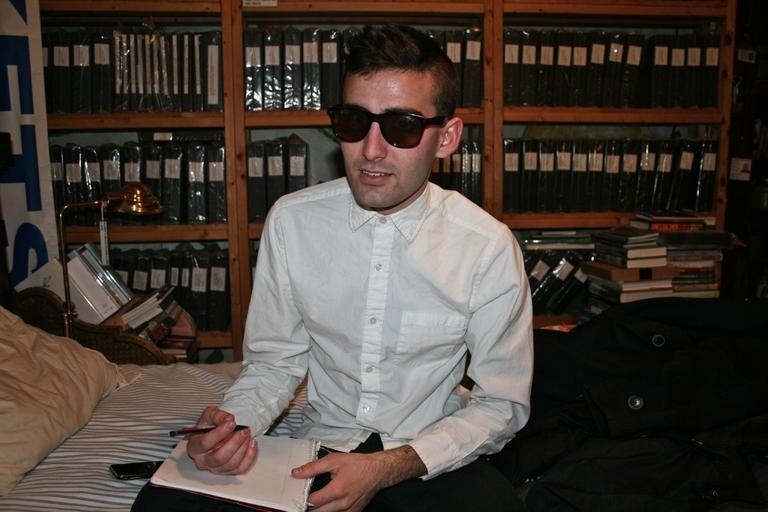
[3, 297, 766, 511]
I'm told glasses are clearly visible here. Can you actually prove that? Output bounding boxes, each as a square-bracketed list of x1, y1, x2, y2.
[326, 104, 442, 149]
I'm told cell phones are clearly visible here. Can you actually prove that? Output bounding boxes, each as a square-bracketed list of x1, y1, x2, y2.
[109, 460, 164, 480]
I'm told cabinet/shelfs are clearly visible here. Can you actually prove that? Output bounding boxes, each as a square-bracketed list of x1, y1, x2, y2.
[239, 3, 494, 362]
[494, 1, 734, 330]
[40, 1, 239, 347]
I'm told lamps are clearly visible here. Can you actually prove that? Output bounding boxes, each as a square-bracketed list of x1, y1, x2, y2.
[57, 182, 168, 316]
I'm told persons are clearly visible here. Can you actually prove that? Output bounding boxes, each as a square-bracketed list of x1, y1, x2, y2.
[128, 27, 535, 511]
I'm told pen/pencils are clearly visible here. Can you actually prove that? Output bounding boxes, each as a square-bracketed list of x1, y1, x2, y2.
[168, 424, 249, 437]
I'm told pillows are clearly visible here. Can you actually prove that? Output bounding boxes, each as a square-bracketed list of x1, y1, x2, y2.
[2, 303, 148, 504]
